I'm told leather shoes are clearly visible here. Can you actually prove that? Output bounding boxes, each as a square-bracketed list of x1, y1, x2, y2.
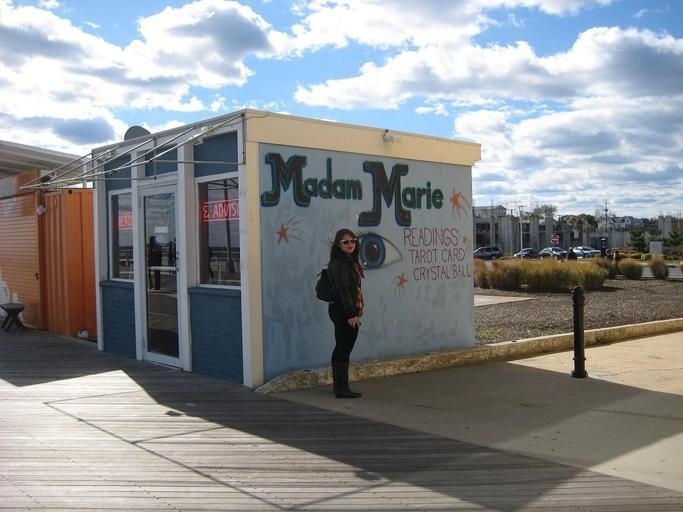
[335, 390, 360, 398]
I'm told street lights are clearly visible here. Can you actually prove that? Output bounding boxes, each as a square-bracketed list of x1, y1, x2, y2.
[516, 205, 526, 259]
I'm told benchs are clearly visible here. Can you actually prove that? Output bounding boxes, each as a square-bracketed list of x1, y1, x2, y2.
[0, 303, 25, 332]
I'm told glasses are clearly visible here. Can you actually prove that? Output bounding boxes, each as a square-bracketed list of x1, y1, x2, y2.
[339, 238, 356, 245]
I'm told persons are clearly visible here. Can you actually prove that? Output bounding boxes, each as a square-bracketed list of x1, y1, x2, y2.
[147, 236, 161, 290]
[528, 248, 539, 259]
[327, 228, 365, 398]
[566, 249, 577, 260]
[600, 246, 622, 275]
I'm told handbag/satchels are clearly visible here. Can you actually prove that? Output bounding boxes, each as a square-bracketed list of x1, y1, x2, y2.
[314, 268, 338, 301]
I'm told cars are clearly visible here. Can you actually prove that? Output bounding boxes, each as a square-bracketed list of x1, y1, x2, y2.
[472, 245, 503, 260]
[513, 247, 540, 260]
[538, 246, 568, 260]
[571, 246, 601, 258]
